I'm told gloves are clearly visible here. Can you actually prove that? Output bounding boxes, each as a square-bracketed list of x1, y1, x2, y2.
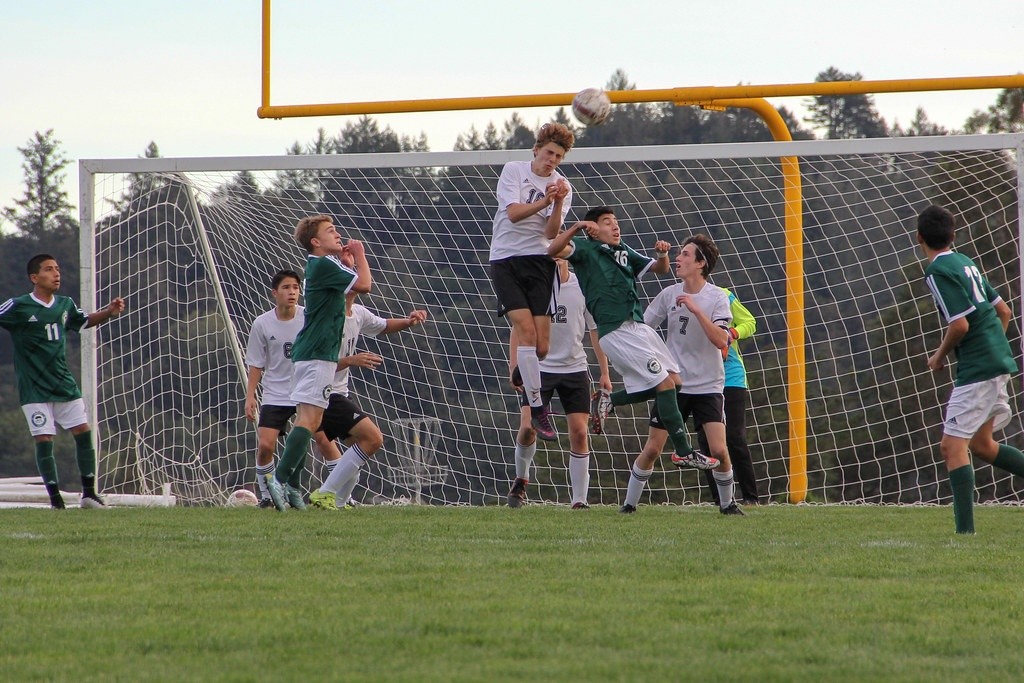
[721, 334, 734, 362]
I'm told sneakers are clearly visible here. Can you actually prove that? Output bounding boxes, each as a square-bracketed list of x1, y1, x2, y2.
[81, 494, 108, 509]
[339, 504, 352, 509]
[571, 502, 590, 509]
[284, 482, 305, 510]
[263, 473, 286, 512]
[531, 410, 560, 441]
[258, 498, 276, 510]
[589, 389, 613, 436]
[513, 364, 525, 387]
[51, 501, 66, 511]
[346, 497, 363, 507]
[308, 488, 338, 510]
[619, 504, 637, 514]
[746, 499, 760, 509]
[670, 448, 720, 471]
[719, 501, 744, 516]
[509, 479, 528, 509]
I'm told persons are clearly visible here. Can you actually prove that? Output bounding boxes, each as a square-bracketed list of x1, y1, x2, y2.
[693, 284, 759, 508]
[546, 206, 723, 469]
[262, 212, 370, 509]
[618, 234, 748, 515]
[507, 230, 614, 509]
[310, 289, 427, 511]
[0, 253, 125, 511]
[916, 204, 1024, 537]
[489, 123, 574, 440]
[244, 270, 357, 511]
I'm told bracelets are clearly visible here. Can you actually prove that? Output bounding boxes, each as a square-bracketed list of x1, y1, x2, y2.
[656, 251, 669, 258]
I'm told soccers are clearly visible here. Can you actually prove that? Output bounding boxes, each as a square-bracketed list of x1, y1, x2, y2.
[573, 87, 611, 126]
